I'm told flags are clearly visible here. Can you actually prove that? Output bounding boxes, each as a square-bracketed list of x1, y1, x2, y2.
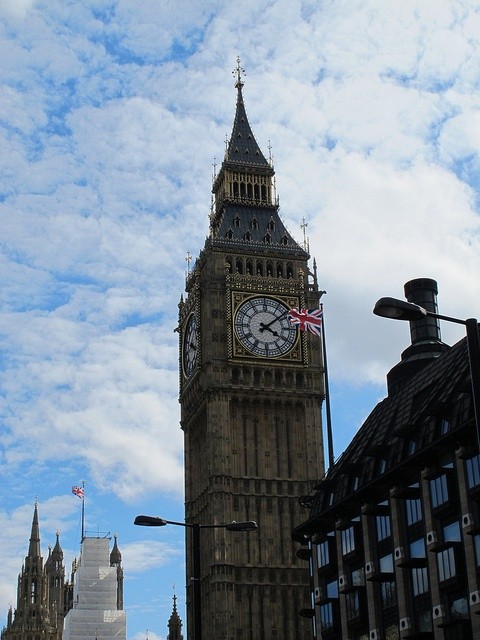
[287, 307, 322, 337]
[72, 486, 84, 499]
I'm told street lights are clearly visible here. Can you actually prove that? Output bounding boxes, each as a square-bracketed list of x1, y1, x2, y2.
[134, 515, 259, 640]
[373, 297, 479, 445]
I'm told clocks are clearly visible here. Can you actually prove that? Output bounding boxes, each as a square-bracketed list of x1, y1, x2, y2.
[225, 281, 309, 369]
[178, 301, 201, 381]
